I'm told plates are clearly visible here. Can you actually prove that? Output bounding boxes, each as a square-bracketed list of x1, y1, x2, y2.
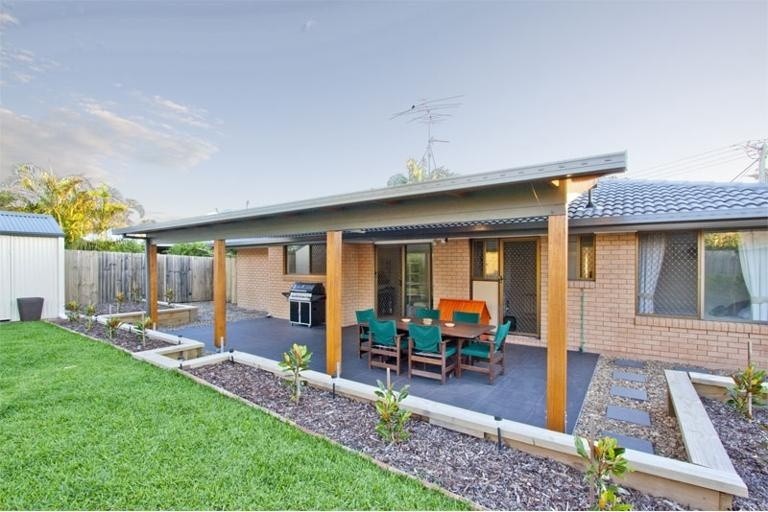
[445, 323, 455, 328]
[401, 318, 411, 323]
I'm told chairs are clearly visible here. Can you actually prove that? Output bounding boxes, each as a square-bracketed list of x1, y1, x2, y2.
[354, 308, 512, 386]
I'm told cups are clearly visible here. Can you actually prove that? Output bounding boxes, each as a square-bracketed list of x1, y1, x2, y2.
[424, 319, 433, 325]
